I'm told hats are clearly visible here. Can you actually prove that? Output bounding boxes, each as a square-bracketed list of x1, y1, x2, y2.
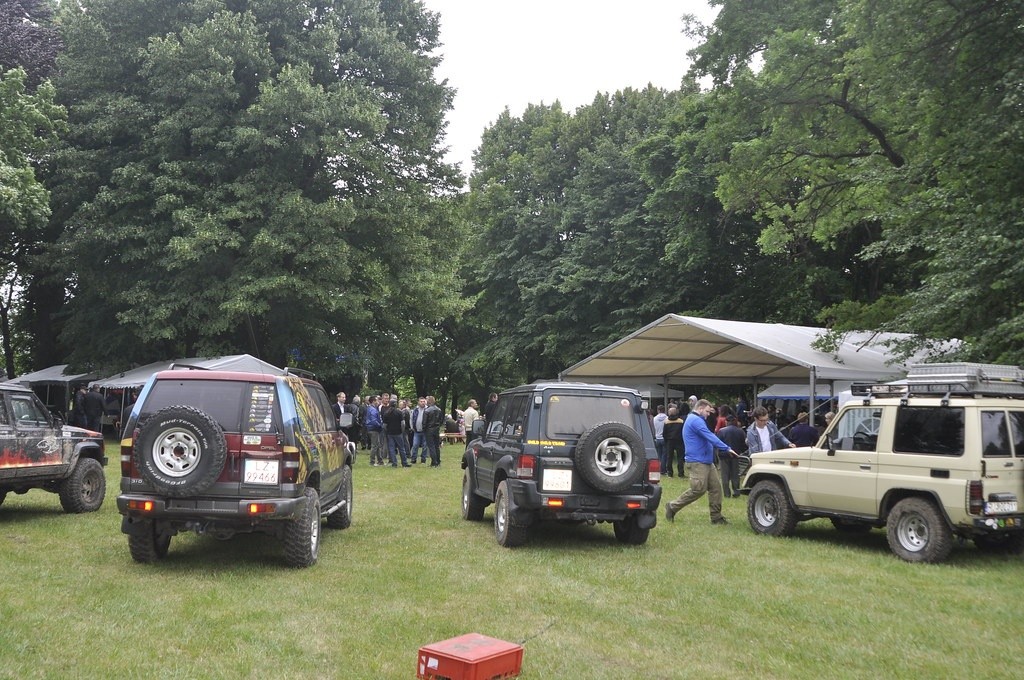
[689, 395, 698, 405]
[389, 399, 397, 405]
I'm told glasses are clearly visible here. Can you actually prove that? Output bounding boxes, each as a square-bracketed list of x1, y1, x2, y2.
[758, 417, 770, 422]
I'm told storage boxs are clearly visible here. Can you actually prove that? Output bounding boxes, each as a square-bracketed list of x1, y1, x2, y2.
[416, 632, 522, 680]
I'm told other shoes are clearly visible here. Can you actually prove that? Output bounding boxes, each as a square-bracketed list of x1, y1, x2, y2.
[732, 493, 740, 497]
[404, 464, 412, 467]
[661, 470, 668, 475]
[712, 517, 731, 526]
[668, 474, 673, 477]
[369, 461, 375, 465]
[378, 459, 384, 465]
[410, 460, 416, 463]
[392, 463, 397, 467]
[678, 474, 686, 478]
[422, 459, 426, 463]
[664, 502, 676, 522]
[428, 464, 437, 468]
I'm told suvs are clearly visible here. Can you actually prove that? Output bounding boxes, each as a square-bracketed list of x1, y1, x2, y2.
[737, 377, 1024, 564]
[461, 382, 662, 547]
[117, 363, 356, 568]
[0, 384, 108, 513]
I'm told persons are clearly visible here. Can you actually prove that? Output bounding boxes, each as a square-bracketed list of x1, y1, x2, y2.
[422, 395, 442, 467]
[653, 395, 827, 478]
[745, 407, 796, 459]
[483, 391, 498, 421]
[465, 398, 480, 451]
[75, 384, 110, 430]
[825, 412, 836, 425]
[121, 386, 144, 433]
[664, 400, 739, 525]
[788, 412, 820, 448]
[716, 415, 745, 497]
[410, 397, 427, 463]
[445, 413, 460, 433]
[332, 391, 414, 467]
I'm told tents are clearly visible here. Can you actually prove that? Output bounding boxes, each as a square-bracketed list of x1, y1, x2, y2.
[631, 384, 684, 408]
[87, 354, 298, 439]
[755, 384, 852, 423]
[557, 313, 974, 426]
[3, 363, 141, 433]
